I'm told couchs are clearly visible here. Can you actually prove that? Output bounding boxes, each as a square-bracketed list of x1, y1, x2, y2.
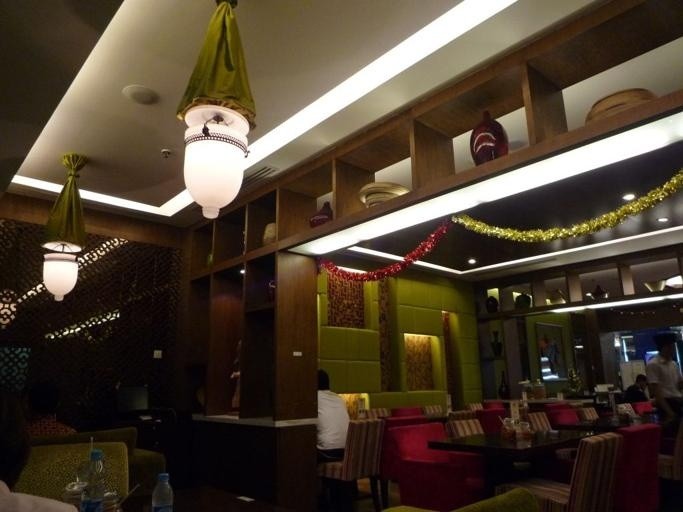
[30, 426, 165, 497]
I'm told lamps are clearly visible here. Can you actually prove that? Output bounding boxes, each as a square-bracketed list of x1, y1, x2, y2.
[176, 0, 257, 220]
[40, 153, 89, 301]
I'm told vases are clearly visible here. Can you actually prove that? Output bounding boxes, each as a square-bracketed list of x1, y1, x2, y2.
[262, 223, 276, 245]
[470, 110, 509, 166]
[310, 201, 332, 228]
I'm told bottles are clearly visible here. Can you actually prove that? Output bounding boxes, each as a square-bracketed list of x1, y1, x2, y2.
[63, 481, 87, 509]
[104, 492, 124, 512]
[515, 422, 532, 449]
[651, 407, 658, 421]
[80, 449, 105, 512]
[152, 473, 173, 512]
[503, 417, 516, 448]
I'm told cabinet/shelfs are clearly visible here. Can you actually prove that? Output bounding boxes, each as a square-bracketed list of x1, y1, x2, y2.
[474, 243, 683, 400]
[175, 0, 683, 427]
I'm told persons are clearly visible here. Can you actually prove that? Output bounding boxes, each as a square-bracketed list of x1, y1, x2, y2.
[645, 332, 682, 502]
[317, 367, 350, 464]
[567, 359, 585, 395]
[623, 374, 651, 423]
[0, 386, 78, 512]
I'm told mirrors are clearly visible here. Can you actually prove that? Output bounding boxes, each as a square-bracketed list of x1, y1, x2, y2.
[535, 322, 570, 382]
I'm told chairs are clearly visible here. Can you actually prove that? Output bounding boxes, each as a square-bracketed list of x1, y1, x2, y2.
[13, 441, 144, 512]
